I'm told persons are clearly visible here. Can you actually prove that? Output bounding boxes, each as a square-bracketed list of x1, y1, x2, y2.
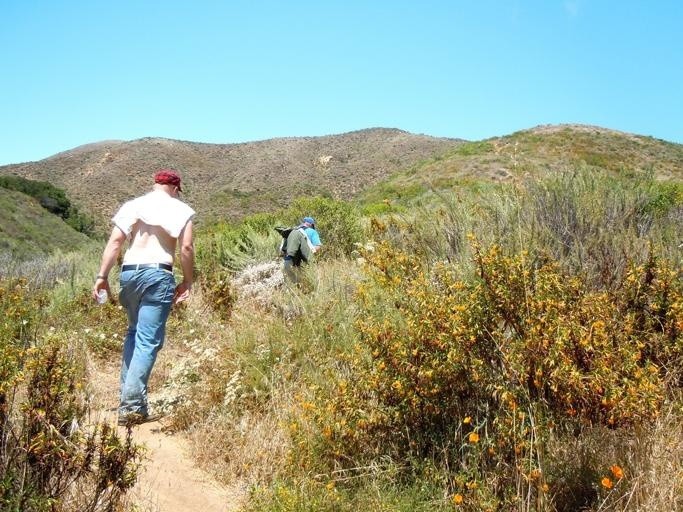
[281, 218, 326, 281]
[92, 169, 199, 428]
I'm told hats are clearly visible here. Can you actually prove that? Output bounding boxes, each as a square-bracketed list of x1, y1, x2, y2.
[304, 217, 315, 229]
[154, 170, 182, 192]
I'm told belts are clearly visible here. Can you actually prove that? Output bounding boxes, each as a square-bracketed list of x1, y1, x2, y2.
[122, 264, 172, 270]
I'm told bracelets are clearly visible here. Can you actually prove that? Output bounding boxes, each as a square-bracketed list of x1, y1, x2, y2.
[95, 275, 108, 279]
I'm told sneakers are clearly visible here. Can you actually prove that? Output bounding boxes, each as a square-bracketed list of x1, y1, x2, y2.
[118, 414, 159, 426]
